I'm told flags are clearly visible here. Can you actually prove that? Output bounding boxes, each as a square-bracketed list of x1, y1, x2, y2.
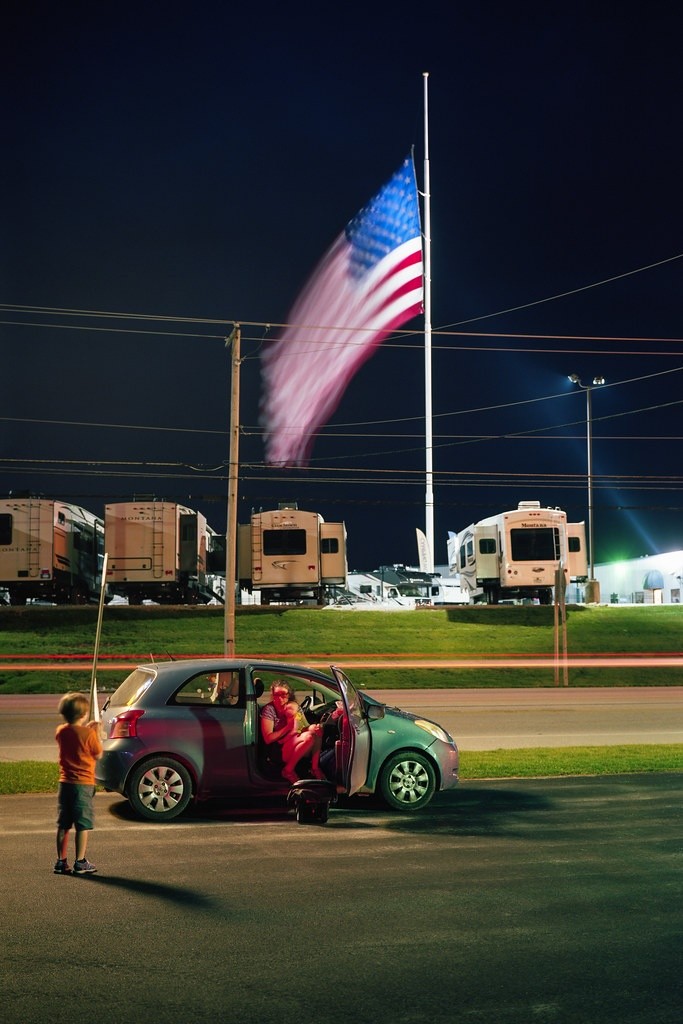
[260, 143, 425, 471]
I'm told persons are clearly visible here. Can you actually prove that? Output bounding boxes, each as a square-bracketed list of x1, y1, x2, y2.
[261, 679, 324, 784]
[53, 692, 104, 873]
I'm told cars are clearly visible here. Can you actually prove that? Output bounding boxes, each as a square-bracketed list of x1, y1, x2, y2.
[96, 656, 463, 812]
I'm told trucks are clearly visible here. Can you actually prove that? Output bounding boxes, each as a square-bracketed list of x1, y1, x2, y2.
[236, 506, 350, 603]
[447, 500, 590, 610]
[101, 500, 225, 603]
[1, 497, 113, 603]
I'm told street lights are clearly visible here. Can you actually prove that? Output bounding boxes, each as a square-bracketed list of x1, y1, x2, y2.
[566, 372, 607, 607]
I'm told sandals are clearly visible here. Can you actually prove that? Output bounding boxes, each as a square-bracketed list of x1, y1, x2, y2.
[73, 858, 97, 873]
[54, 861, 73, 874]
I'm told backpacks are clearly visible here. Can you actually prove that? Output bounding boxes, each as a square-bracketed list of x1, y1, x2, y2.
[287, 779, 338, 803]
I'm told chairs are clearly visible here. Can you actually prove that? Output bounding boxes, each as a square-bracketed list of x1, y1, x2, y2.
[254, 678, 263, 697]
[223, 678, 239, 705]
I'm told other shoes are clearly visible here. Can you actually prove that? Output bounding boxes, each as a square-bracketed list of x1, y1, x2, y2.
[311, 768, 325, 779]
[281, 768, 300, 784]
